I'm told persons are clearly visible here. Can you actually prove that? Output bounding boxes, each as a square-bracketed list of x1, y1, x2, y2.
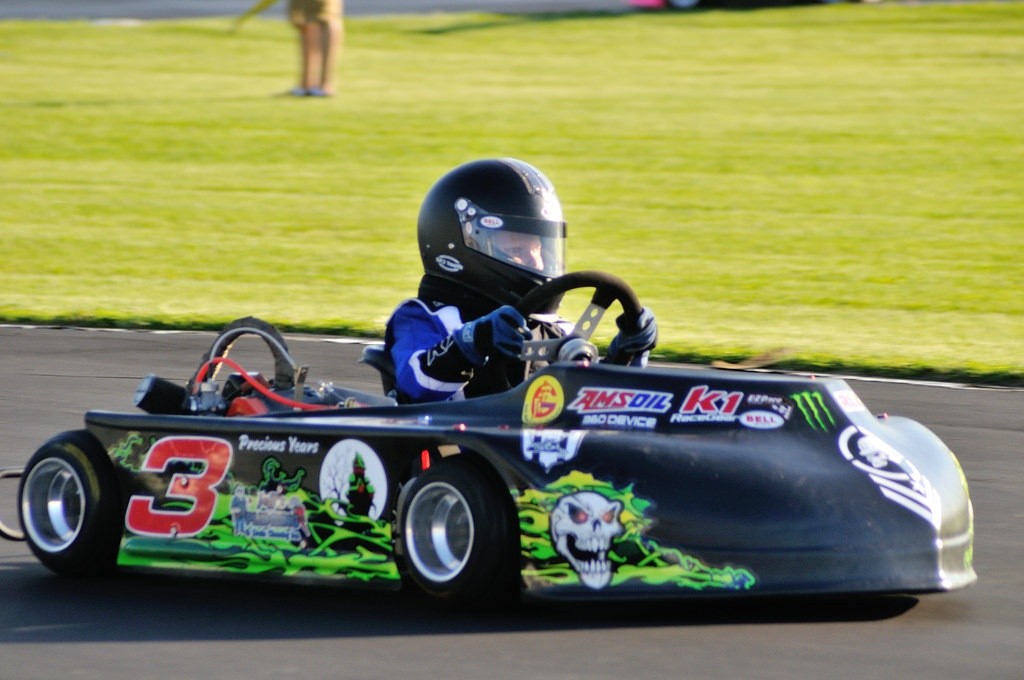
[385, 158, 657, 407]
[280, 0, 338, 97]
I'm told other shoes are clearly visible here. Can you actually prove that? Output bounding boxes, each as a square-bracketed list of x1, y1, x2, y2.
[289, 87, 324, 96]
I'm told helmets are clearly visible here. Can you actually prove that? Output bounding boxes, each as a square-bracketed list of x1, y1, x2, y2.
[417, 157, 568, 332]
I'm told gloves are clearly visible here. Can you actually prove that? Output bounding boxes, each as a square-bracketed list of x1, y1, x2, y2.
[607, 307, 658, 365]
[453, 305, 533, 366]
